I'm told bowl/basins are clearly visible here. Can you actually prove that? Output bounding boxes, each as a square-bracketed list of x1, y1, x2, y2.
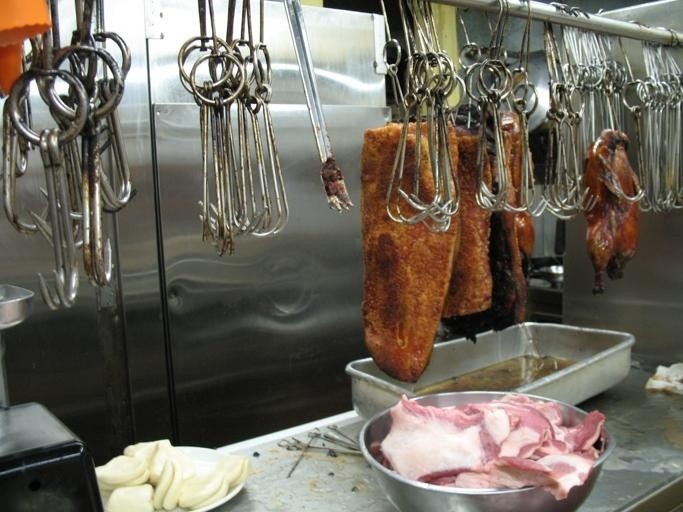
[358, 390, 619, 511]
[539, 265, 564, 288]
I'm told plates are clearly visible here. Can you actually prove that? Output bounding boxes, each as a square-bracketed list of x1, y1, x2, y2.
[172, 446, 249, 512]
